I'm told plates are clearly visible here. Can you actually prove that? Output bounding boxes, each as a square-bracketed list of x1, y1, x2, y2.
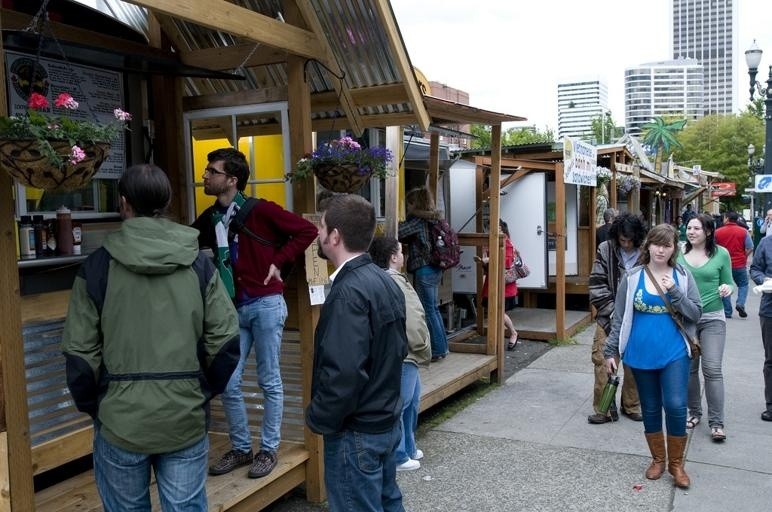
[763, 289, 772, 293]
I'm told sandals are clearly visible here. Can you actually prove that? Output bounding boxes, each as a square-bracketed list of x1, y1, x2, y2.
[686, 415, 699, 428]
[712, 427, 726, 438]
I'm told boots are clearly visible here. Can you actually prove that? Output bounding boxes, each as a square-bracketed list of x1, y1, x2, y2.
[667, 434, 690, 487]
[645, 431, 665, 480]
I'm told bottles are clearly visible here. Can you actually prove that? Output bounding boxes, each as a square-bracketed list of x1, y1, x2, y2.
[514, 259, 529, 277]
[32, 215, 49, 260]
[14, 215, 20, 261]
[434, 235, 445, 255]
[20, 215, 39, 261]
[71, 222, 84, 256]
[55, 204, 75, 258]
[44, 218, 60, 259]
[598, 373, 621, 417]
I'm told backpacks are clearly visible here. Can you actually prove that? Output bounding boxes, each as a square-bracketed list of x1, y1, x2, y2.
[411, 216, 463, 285]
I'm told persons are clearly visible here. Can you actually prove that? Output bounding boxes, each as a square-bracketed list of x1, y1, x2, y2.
[184, 149, 321, 481]
[471, 217, 519, 354]
[303, 194, 407, 510]
[62, 163, 241, 511]
[372, 230, 433, 473]
[398, 187, 450, 363]
[590, 202, 771, 487]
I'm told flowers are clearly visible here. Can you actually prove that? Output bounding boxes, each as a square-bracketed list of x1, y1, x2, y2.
[617, 174, 641, 194]
[595, 165, 614, 183]
[0, 88, 134, 167]
[278, 136, 393, 183]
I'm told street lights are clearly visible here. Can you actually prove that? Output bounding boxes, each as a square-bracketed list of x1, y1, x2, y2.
[743, 37, 772, 220]
[747, 142, 766, 218]
[747, 156, 764, 216]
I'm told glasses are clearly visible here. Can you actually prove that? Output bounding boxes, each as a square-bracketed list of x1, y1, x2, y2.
[205, 168, 234, 177]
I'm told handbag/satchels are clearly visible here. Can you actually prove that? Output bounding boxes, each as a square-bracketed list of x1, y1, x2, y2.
[690, 336, 702, 358]
[504, 252, 529, 284]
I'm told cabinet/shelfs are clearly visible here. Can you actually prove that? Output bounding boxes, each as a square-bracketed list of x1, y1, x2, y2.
[15, 253, 96, 486]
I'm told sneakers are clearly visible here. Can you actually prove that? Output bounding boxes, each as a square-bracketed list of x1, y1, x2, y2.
[621, 405, 642, 421]
[248, 450, 277, 477]
[736, 306, 747, 317]
[396, 449, 424, 471]
[588, 410, 618, 423]
[209, 448, 253, 474]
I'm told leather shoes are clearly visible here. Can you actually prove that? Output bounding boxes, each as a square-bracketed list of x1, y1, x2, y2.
[508, 334, 519, 349]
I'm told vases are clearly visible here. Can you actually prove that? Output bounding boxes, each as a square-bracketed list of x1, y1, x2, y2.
[312, 164, 375, 194]
[0, 138, 108, 195]
